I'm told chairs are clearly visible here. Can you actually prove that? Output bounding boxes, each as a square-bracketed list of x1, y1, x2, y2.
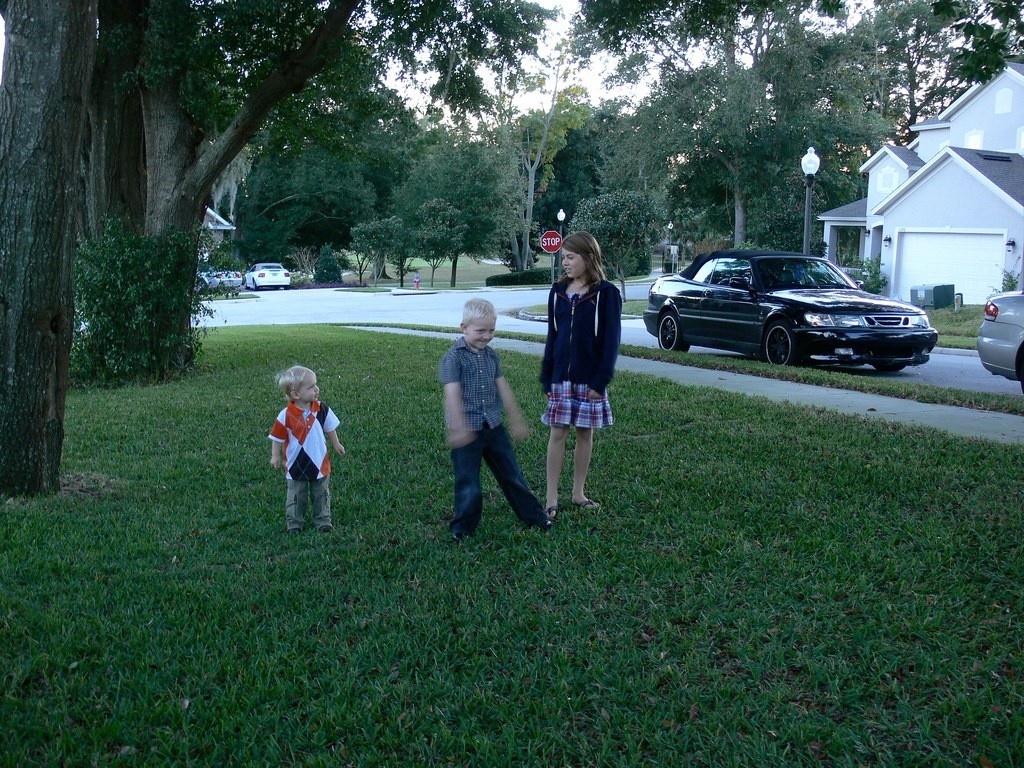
[779, 270, 794, 281]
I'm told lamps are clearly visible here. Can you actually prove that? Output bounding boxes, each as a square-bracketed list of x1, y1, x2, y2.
[883, 235, 892, 248]
[1005, 237, 1016, 252]
[864, 229, 870, 238]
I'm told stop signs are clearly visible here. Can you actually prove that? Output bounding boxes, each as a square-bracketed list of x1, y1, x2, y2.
[539, 230, 563, 254]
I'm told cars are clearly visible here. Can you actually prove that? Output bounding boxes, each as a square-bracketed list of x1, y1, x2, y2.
[243, 262, 291, 291]
[194, 264, 243, 294]
[643, 248, 940, 372]
[976, 290, 1024, 395]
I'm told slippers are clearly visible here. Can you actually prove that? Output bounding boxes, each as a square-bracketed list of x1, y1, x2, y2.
[572, 496, 600, 512]
[545, 502, 559, 518]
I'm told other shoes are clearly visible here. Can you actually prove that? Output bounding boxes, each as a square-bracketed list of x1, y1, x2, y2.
[454, 532, 469, 542]
[289, 529, 299, 535]
[320, 525, 330, 532]
[539, 517, 558, 530]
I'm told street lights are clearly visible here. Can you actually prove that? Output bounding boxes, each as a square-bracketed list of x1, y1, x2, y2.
[667, 221, 674, 255]
[800, 146, 821, 255]
[556, 209, 566, 281]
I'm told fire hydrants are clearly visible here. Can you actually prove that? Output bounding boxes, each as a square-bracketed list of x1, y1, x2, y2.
[413, 275, 420, 288]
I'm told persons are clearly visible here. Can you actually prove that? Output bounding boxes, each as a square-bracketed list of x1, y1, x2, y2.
[441, 298, 552, 544]
[267, 366, 346, 535]
[539, 231, 621, 521]
